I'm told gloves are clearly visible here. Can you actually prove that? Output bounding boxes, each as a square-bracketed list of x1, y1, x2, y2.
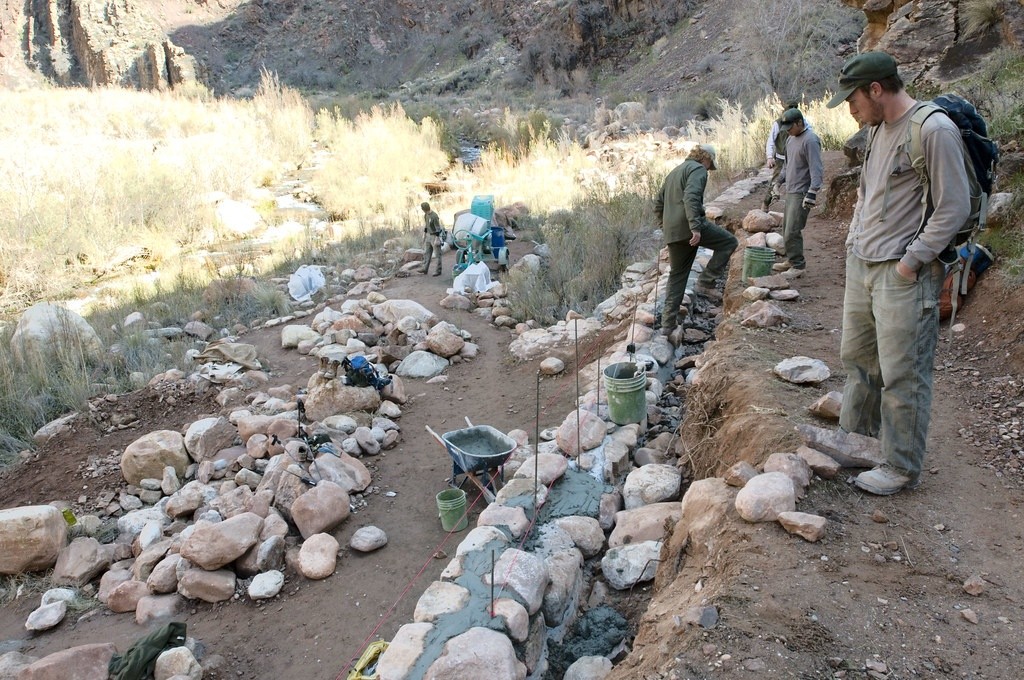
[802, 190, 817, 209]
[767, 158, 776, 169]
[770, 181, 781, 202]
[434, 237, 440, 247]
[422, 232, 426, 242]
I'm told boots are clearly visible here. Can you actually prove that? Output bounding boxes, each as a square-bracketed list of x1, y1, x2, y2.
[323, 360, 340, 379]
[318, 356, 330, 377]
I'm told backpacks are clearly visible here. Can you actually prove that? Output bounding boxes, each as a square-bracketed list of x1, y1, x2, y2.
[864, 90, 1000, 264]
[342, 355, 391, 388]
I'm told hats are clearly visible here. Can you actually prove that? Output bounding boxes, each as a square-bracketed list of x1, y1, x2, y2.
[825, 52, 897, 109]
[696, 143, 717, 170]
[779, 108, 803, 131]
[785, 99, 798, 110]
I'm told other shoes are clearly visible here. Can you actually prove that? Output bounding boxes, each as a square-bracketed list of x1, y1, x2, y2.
[693, 283, 723, 300]
[662, 319, 678, 334]
[761, 202, 769, 214]
[773, 261, 792, 271]
[433, 270, 441, 276]
[855, 464, 921, 494]
[417, 269, 428, 274]
[781, 268, 806, 280]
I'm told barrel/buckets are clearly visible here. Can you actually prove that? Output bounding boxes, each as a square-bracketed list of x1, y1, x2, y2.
[436, 488, 468, 533]
[603, 361, 648, 426]
[742, 245, 777, 288]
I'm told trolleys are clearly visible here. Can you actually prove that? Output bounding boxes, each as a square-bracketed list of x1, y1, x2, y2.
[425, 418, 516, 505]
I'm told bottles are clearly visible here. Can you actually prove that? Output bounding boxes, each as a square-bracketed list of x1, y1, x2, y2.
[61, 507, 77, 525]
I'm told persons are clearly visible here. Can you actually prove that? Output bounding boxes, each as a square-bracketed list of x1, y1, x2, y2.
[827, 52, 973, 497]
[761, 100, 814, 213]
[770, 109, 824, 278]
[416, 202, 443, 276]
[653, 143, 739, 335]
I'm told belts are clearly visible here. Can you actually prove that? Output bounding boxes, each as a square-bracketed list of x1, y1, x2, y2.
[775, 153, 786, 159]
